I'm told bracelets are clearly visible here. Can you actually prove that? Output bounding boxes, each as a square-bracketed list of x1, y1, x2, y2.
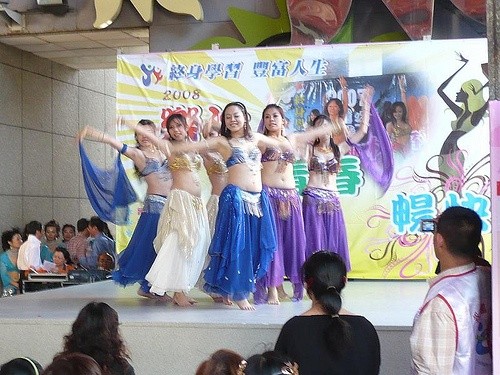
[90, 128, 105, 143]
[358, 104, 372, 127]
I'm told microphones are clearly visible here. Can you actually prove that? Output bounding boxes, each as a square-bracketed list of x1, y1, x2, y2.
[77, 262, 89, 272]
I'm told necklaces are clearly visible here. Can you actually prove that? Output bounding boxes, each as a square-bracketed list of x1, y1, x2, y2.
[271, 134, 285, 142]
[10, 248, 18, 256]
[231, 136, 246, 145]
[151, 144, 155, 152]
[316, 143, 333, 153]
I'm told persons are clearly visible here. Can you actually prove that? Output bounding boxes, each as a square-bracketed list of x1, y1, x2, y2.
[409, 205, 493, 375]
[0, 216, 116, 295]
[66, 300, 134, 375]
[302, 83, 375, 293]
[78, 118, 175, 304]
[274, 249, 381, 375]
[117, 111, 234, 307]
[307, 74, 412, 156]
[158, 102, 300, 311]
[253, 104, 350, 306]
